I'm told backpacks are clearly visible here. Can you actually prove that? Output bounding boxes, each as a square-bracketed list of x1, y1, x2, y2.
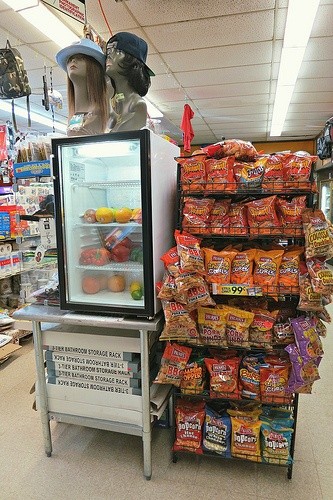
[316, 116, 332, 164]
[0, 39, 31, 133]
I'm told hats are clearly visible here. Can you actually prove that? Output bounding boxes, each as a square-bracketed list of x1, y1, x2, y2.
[105, 32, 156, 76]
[56, 38, 106, 73]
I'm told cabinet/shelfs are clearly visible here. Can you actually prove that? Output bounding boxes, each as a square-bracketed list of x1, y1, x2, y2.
[167, 134, 320, 481]
[0, 117, 55, 364]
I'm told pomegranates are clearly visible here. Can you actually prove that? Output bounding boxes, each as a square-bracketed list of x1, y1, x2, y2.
[82, 274, 125, 293]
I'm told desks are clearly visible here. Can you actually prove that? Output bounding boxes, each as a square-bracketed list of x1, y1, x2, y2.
[9, 303, 180, 480]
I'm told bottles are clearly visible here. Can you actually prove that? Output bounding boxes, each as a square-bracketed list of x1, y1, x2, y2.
[0, 125, 7, 158]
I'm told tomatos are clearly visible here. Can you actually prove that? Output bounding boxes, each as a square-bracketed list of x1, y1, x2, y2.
[79, 248, 110, 266]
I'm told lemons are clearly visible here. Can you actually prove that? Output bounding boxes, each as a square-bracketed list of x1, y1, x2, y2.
[129, 281, 144, 299]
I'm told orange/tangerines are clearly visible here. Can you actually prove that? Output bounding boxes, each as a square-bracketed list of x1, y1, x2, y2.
[94, 207, 114, 223]
[115, 208, 132, 223]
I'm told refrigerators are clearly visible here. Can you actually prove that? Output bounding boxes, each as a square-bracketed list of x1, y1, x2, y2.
[51, 129, 181, 316]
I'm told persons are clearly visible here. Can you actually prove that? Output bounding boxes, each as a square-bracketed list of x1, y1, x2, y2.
[55, 39, 111, 136]
[105, 32, 147, 133]
